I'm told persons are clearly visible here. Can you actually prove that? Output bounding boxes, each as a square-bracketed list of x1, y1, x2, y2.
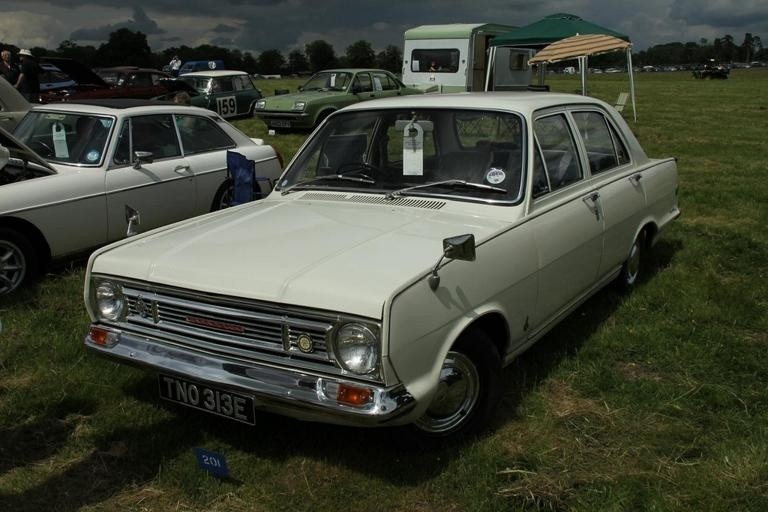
[169, 55, 182, 78]
[0, 50, 19, 86]
[429, 60, 442, 72]
[171, 91, 211, 149]
[717, 63, 726, 78]
[13, 48, 40, 103]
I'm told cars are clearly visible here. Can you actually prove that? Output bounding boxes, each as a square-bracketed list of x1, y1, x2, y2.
[0, 97, 287, 311]
[254, 66, 436, 138]
[554, 57, 765, 81]
[87, 88, 681, 456]
